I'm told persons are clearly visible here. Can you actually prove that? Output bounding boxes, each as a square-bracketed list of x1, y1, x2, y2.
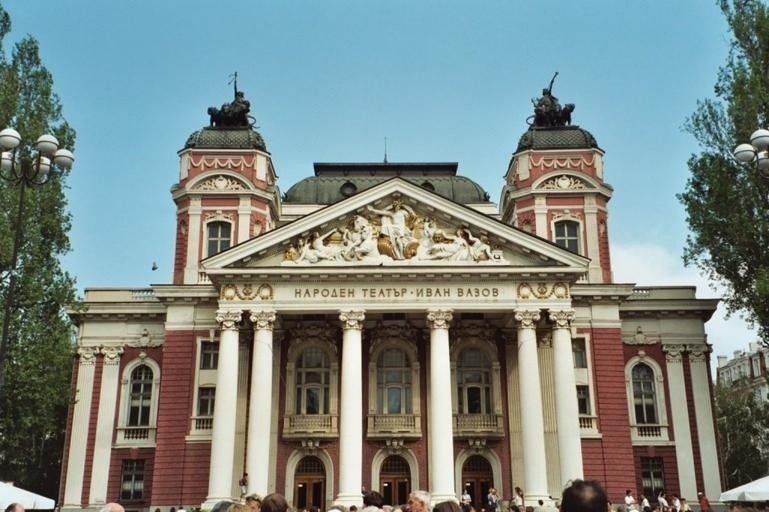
[154, 473, 768, 512]
[5, 503, 25, 512]
[535, 89, 577, 125]
[100, 502, 125, 512]
[286, 197, 496, 263]
[208, 88, 251, 126]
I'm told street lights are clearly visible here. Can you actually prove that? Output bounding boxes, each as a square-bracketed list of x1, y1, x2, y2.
[733, 129, 769, 179]
[0, 128, 75, 388]
